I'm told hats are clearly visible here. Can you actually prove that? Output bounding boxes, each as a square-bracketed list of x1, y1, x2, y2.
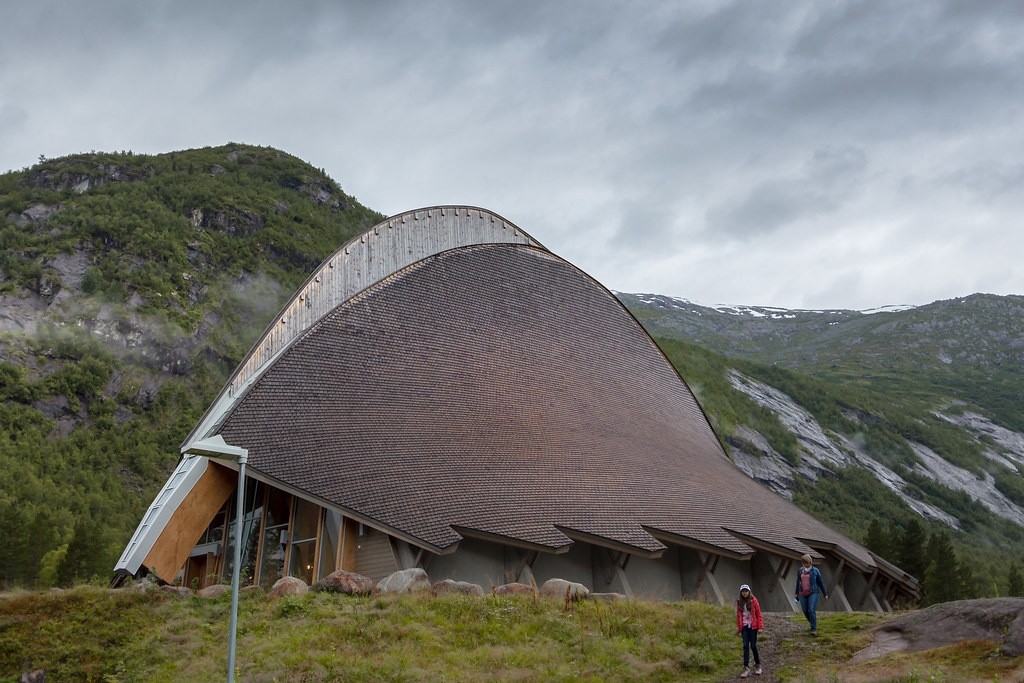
[740, 584, 750, 591]
[801, 555, 812, 563]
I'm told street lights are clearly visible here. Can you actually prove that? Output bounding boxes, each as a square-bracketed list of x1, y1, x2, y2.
[180, 433, 250, 683]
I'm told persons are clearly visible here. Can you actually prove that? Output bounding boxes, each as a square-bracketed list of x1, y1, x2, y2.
[794, 554, 829, 636]
[736, 583, 768, 677]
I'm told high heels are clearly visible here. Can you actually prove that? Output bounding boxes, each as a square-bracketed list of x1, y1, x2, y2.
[741, 667, 751, 677]
[754, 662, 762, 674]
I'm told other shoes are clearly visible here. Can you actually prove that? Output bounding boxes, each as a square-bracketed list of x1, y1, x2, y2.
[811, 631, 816, 634]
